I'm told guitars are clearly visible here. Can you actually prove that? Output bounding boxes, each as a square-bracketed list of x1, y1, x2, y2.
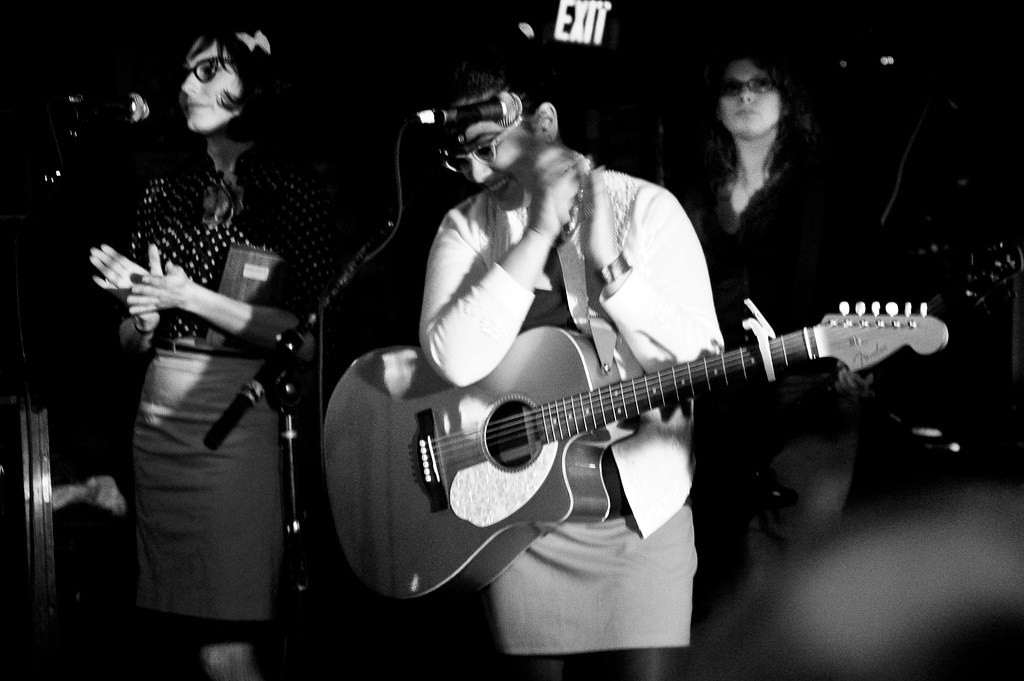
[321, 298, 950, 604]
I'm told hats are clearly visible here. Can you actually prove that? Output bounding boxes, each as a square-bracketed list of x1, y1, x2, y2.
[215, 23, 280, 104]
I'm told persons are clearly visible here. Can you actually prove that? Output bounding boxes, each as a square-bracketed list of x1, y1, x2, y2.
[87, 21, 350, 681]
[419, 45, 724, 681]
[667, 42, 899, 625]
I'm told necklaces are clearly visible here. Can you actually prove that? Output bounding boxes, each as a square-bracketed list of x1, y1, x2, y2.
[562, 165, 586, 239]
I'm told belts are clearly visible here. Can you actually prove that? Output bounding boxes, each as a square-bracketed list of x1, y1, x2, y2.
[157, 339, 263, 360]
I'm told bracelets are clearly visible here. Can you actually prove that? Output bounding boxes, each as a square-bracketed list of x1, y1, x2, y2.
[595, 251, 632, 285]
[526, 224, 553, 241]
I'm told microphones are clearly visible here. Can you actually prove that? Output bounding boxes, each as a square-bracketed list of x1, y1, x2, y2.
[415, 91, 523, 128]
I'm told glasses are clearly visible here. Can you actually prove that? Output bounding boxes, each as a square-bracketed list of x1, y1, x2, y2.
[443, 116, 523, 172]
[177, 58, 232, 84]
[719, 78, 774, 96]
